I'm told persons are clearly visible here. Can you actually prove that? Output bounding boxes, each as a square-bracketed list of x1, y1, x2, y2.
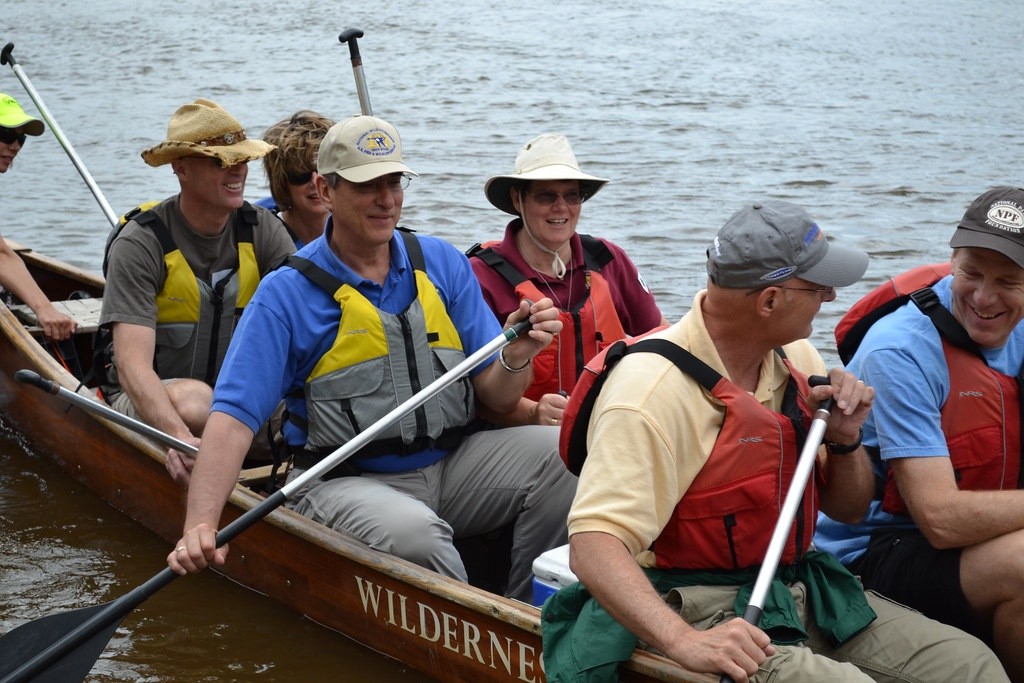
[465, 129, 673, 426]
[98, 97, 299, 491]
[262, 109, 337, 250]
[811, 186, 1024, 683]
[0, 93, 78, 340]
[168, 115, 578, 606]
[542, 199, 1011, 683]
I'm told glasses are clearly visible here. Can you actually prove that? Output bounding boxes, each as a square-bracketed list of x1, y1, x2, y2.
[340, 173, 412, 193]
[0, 126, 27, 148]
[185, 156, 250, 169]
[523, 188, 586, 205]
[745, 285, 833, 298]
[289, 169, 318, 185]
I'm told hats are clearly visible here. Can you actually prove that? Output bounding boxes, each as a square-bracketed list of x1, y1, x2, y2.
[141, 98, 278, 168]
[950, 187, 1024, 270]
[484, 133, 609, 216]
[317, 115, 419, 182]
[0, 93, 45, 137]
[705, 201, 868, 291]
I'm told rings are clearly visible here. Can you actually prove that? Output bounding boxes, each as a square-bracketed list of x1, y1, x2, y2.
[176, 546, 187, 552]
[551, 419, 557, 426]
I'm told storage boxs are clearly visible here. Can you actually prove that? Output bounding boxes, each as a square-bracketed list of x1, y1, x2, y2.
[532, 544, 579, 607]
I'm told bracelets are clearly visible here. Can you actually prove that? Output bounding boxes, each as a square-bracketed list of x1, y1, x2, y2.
[499, 348, 531, 373]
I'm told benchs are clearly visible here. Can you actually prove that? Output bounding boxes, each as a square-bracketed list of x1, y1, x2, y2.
[7, 298, 103, 334]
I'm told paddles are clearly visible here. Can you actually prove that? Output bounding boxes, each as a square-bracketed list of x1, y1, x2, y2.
[0, 299, 534, 683]
[720, 375, 835, 683]
[0, 42, 118, 227]
[14, 369, 198, 458]
[339, 28, 373, 116]
